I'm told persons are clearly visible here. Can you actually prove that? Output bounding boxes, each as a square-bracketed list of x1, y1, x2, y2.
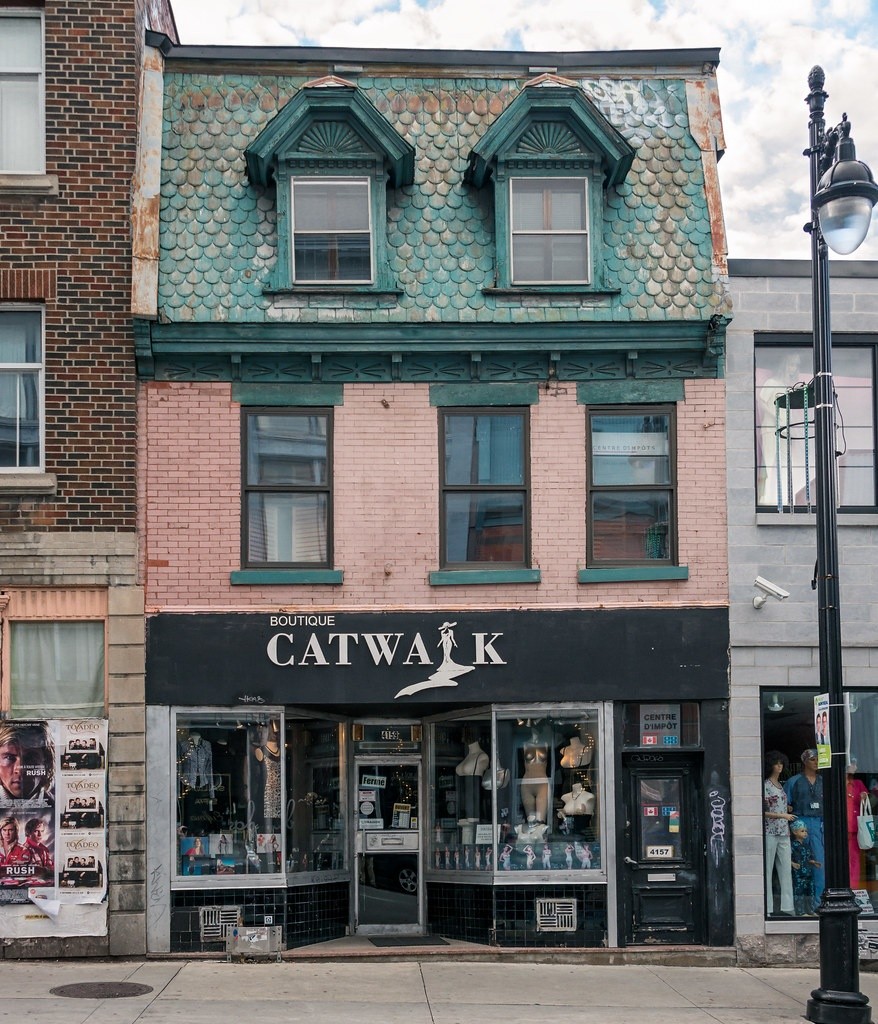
[59, 856, 103, 887]
[523, 845, 536, 870]
[521, 728, 549, 823]
[61, 738, 105, 770]
[186, 838, 204, 854]
[565, 845, 574, 869]
[436, 821, 441, 844]
[542, 845, 552, 870]
[179, 734, 213, 833]
[764, 751, 797, 916]
[0, 818, 51, 864]
[845, 754, 868, 890]
[60, 797, 104, 829]
[0, 719, 55, 804]
[783, 749, 826, 917]
[219, 835, 229, 854]
[560, 737, 594, 843]
[257, 834, 279, 852]
[499, 844, 513, 870]
[815, 711, 828, 744]
[436, 846, 492, 870]
[188, 856, 196, 875]
[456, 741, 511, 823]
[581, 844, 592, 869]
[256, 740, 280, 833]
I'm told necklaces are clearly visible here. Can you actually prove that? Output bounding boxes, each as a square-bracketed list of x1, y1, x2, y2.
[572, 791, 581, 801]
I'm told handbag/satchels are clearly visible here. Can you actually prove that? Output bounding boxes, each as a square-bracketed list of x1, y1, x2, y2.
[857, 792, 878, 849]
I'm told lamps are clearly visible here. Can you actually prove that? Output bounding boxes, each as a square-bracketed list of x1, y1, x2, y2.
[767, 693, 784, 711]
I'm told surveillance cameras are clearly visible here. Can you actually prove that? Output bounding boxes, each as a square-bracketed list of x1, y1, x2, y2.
[754, 576, 790, 601]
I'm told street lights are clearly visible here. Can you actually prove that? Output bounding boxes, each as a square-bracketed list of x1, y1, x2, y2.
[803, 64, 878, 1024]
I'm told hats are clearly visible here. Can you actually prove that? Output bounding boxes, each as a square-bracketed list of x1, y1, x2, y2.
[790, 820, 807, 832]
[801, 750, 818, 764]
